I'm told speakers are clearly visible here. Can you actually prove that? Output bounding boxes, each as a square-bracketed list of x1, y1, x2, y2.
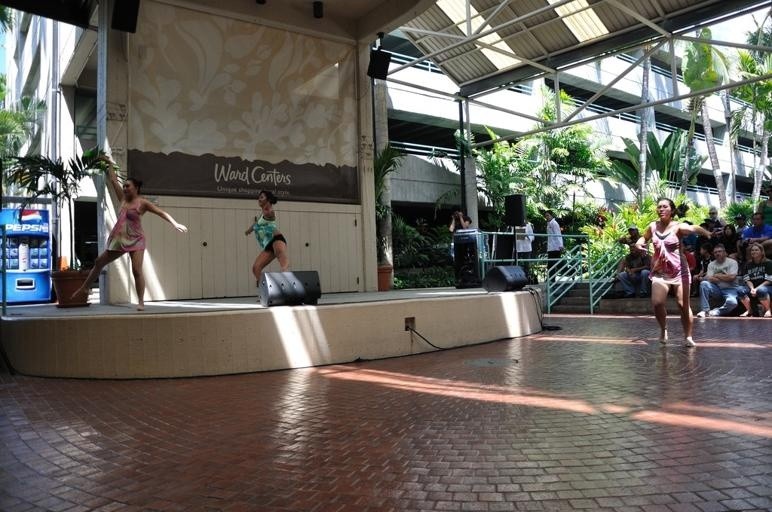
[455, 232, 483, 289]
[505, 195, 526, 226]
[483, 266, 529, 291]
[111, 0, 141, 33]
[259, 271, 322, 307]
[367, 50, 391, 80]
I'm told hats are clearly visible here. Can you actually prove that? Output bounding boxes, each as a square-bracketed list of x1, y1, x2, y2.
[627, 225, 637, 230]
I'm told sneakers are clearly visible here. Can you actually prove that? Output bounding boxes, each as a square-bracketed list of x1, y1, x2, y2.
[739, 310, 754, 317]
[685, 336, 695, 347]
[696, 310, 718, 317]
[640, 293, 648, 298]
[660, 328, 668, 343]
[624, 293, 635, 298]
[764, 310, 772, 317]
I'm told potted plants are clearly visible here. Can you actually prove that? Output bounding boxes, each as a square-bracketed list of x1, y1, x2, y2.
[3, 144, 129, 308]
[374, 140, 408, 291]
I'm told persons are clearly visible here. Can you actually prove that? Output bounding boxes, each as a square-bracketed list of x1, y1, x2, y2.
[544, 210, 575, 282]
[617, 198, 772, 347]
[71, 157, 188, 311]
[245, 191, 289, 302]
[511, 221, 535, 277]
[449, 205, 471, 261]
